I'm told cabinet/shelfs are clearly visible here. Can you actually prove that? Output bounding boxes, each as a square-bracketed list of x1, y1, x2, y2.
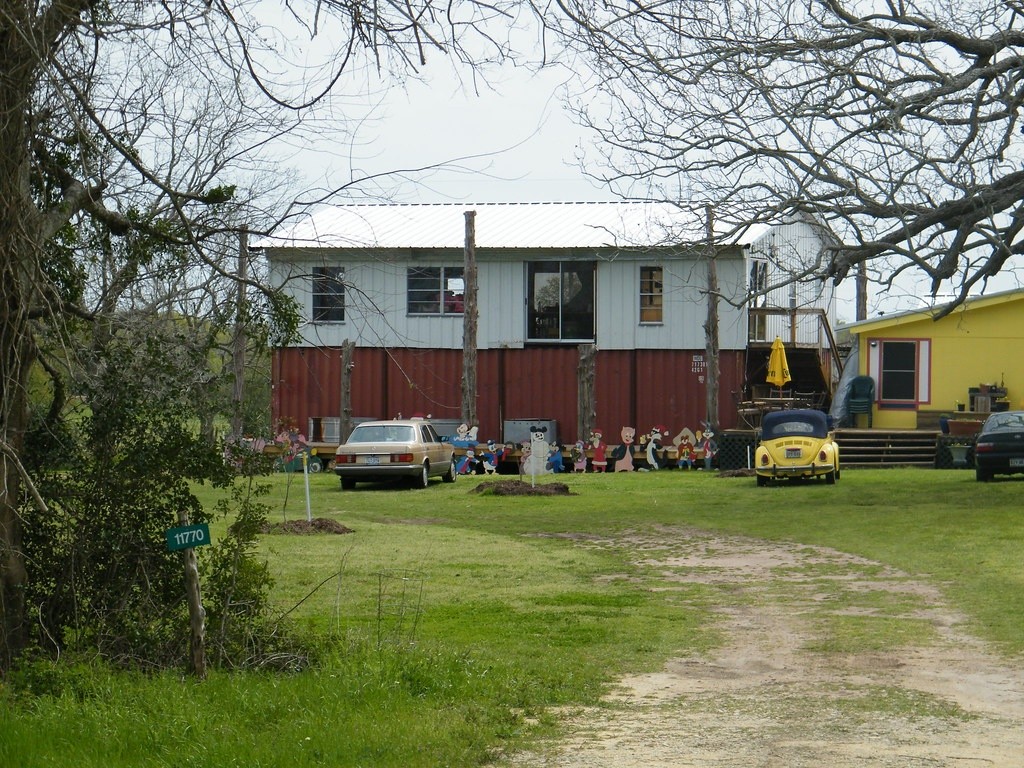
[641, 277, 662, 310]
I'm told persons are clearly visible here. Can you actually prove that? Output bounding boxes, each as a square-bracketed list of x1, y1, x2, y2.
[393, 413, 402, 420]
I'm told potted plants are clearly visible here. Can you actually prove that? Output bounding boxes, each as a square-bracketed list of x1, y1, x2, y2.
[949, 416, 984, 435]
[955, 400, 965, 411]
[946, 443, 972, 463]
[939, 413, 952, 433]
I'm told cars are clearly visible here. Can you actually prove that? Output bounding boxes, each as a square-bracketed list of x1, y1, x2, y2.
[333, 420, 457, 488]
[755, 411, 840, 484]
[973, 410, 1024, 482]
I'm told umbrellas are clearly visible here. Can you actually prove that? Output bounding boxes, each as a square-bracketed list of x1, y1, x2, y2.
[766, 335, 791, 397]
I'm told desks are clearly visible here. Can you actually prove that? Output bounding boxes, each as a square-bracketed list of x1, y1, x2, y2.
[754, 397, 811, 411]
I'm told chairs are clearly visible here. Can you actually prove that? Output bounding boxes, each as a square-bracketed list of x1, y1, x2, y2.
[730, 387, 827, 429]
[845, 374, 876, 427]
[396, 428, 411, 441]
